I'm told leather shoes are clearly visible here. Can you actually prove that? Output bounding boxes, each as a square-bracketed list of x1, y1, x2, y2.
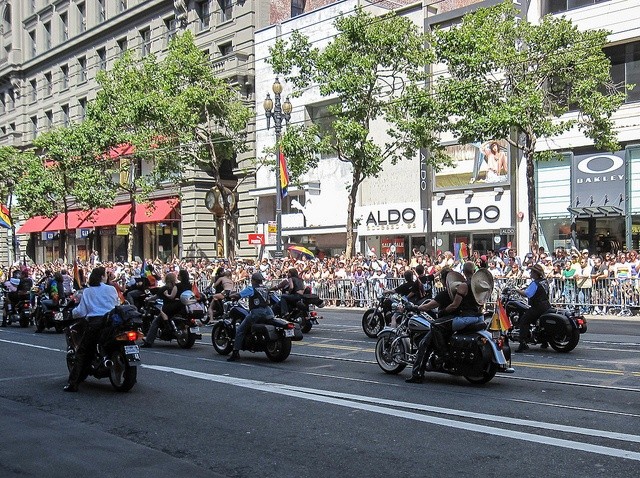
[63, 381, 79, 392]
[226, 349, 240, 361]
[540, 342, 549, 348]
[405, 375, 423, 383]
[514, 342, 529, 352]
[140, 342, 152, 348]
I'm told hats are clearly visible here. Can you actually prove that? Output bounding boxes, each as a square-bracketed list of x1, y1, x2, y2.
[528, 263, 546, 277]
[252, 272, 265, 280]
[471, 268, 494, 305]
[446, 271, 467, 302]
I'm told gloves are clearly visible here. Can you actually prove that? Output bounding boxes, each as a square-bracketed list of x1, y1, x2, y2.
[405, 301, 420, 315]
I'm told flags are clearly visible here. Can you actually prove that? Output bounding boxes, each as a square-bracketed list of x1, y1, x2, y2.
[278, 152, 291, 200]
[0, 202, 12, 230]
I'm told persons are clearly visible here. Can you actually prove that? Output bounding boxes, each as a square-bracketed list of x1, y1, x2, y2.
[0, 257, 74, 273]
[404, 248, 487, 272]
[91, 249, 100, 263]
[28, 270, 51, 315]
[430, 261, 487, 354]
[512, 264, 552, 353]
[383, 270, 414, 326]
[61, 269, 74, 301]
[223, 273, 274, 362]
[403, 265, 458, 391]
[280, 269, 305, 316]
[413, 265, 442, 304]
[478, 140, 508, 185]
[126, 264, 134, 317]
[134, 256, 182, 273]
[64, 267, 119, 391]
[312, 251, 380, 307]
[184, 258, 313, 276]
[40, 273, 63, 317]
[269, 271, 290, 295]
[87, 261, 134, 269]
[75, 255, 85, 266]
[467, 140, 491, 185]
[142, 273, 177, 346]
[160, 268, 193, 336]
[1, 270, 22, 328]
[106, 269, 123, 306]
[487, 247, 640, 281]
[207, 269, 232, 326]
[205, 267, 222, 295]
[8, 269, 34, 317]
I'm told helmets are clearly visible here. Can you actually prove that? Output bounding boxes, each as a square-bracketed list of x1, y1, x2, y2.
[180, 289, 197, 304]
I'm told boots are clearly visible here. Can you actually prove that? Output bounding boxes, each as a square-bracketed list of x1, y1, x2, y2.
[162, 319, 172, 333]
[0, 315, 7, 326]
[213, 299, 224, 317]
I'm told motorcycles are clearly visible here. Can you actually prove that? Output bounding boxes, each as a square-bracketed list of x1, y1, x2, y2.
[1, 289, 30, 326]
[141, 288, 203, 349]
[266, 289, 318, 333]
[33, 293, 74, 332]
[122, 282, 151, 298]
[375, 296, 507, 386]
[199, 291, 232, 324]
[573, 310, 586, 333]
[212, 297, 295, 362]
[67, 309, 141, 392]
[362, 288, 432, 339]
[483, 288, 580, 352]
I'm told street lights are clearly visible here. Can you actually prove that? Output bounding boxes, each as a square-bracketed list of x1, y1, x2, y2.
[264, 77, 292, 257]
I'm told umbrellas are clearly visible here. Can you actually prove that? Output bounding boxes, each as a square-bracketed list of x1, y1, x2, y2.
[289, 242, 316, 259]
